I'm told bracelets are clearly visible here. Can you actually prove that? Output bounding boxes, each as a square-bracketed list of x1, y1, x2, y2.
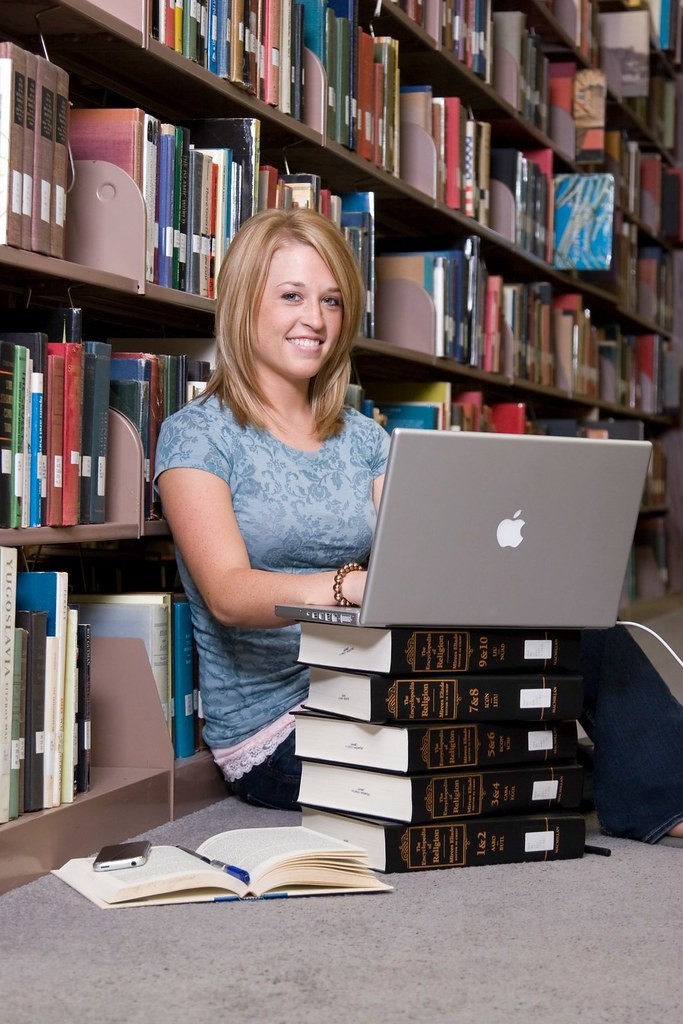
[333, 560, 366, 608]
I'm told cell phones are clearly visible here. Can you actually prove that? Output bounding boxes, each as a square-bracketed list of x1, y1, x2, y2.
[93, 840, 151, 872]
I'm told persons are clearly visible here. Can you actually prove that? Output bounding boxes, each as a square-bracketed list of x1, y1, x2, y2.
[150, 204, 683, 852]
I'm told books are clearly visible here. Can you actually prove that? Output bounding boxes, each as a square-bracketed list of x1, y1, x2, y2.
[0, 0, 683, 533]
[618, 513, 670, 611]
[50, 823, 394, 909]
[287, 616, 588, 875]
[1, 536, 214, 828]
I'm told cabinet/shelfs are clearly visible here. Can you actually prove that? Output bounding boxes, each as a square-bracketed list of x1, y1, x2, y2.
[0, 0, 683, 895]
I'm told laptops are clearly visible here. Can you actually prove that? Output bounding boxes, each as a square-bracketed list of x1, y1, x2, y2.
[276, 428, 654, 630]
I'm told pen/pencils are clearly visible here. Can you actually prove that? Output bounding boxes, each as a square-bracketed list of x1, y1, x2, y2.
[176, 844, 250, 884]
